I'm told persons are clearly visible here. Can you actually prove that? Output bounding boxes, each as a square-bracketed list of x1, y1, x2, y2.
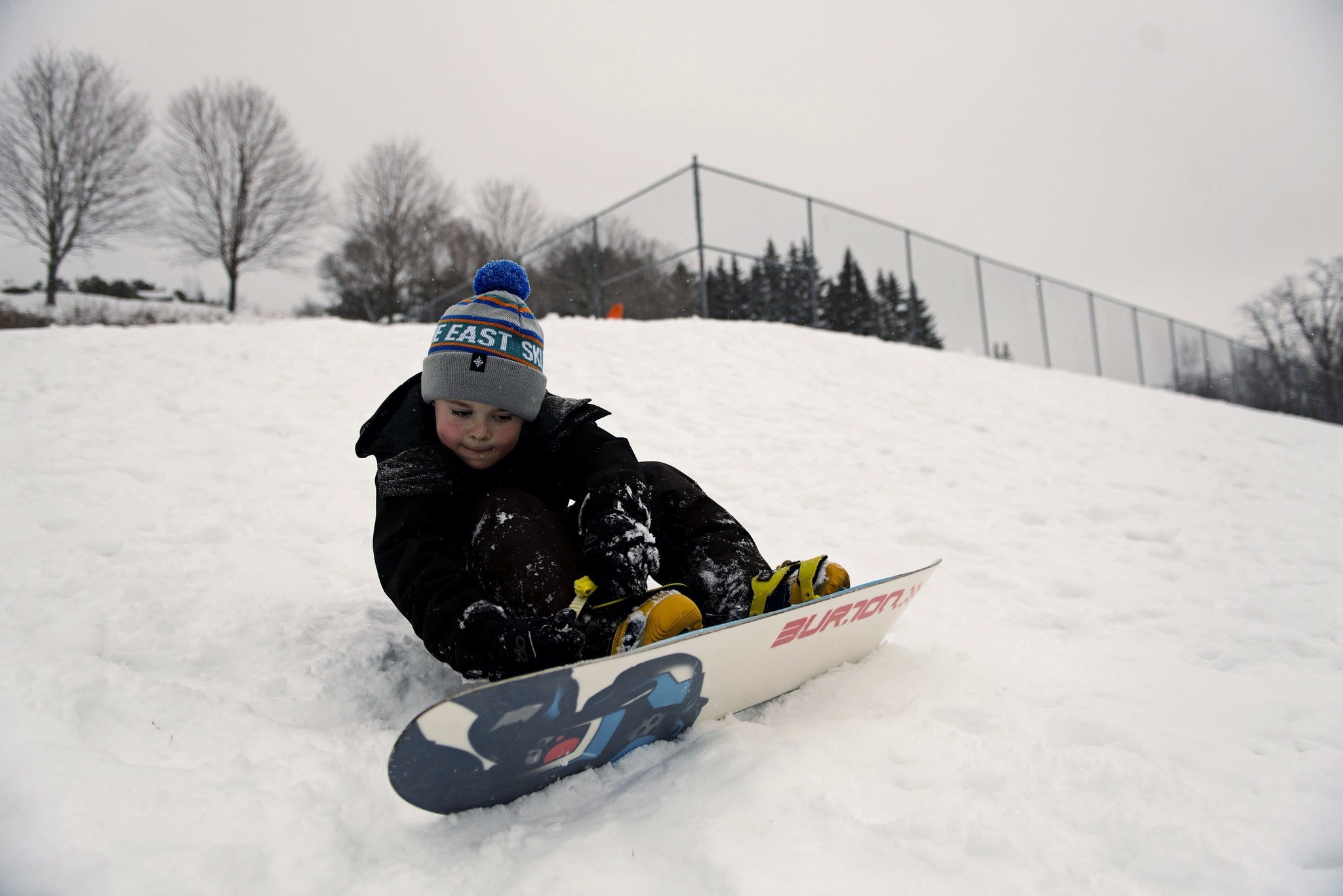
[355, 260, 850, 685]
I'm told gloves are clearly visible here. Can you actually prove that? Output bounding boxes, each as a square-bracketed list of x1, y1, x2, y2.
[458, 606, 584, 669]
[585, 512, 659, 597]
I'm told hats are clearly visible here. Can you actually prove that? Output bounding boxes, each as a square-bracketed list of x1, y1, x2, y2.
[421, 259, 547, 420]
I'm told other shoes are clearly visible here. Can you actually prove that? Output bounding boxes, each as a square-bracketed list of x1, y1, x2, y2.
[607, 592, 704, 655]
[739, 560, 850, 609]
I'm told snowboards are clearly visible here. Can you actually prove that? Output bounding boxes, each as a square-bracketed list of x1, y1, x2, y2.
[389, 558, 943, 818]
